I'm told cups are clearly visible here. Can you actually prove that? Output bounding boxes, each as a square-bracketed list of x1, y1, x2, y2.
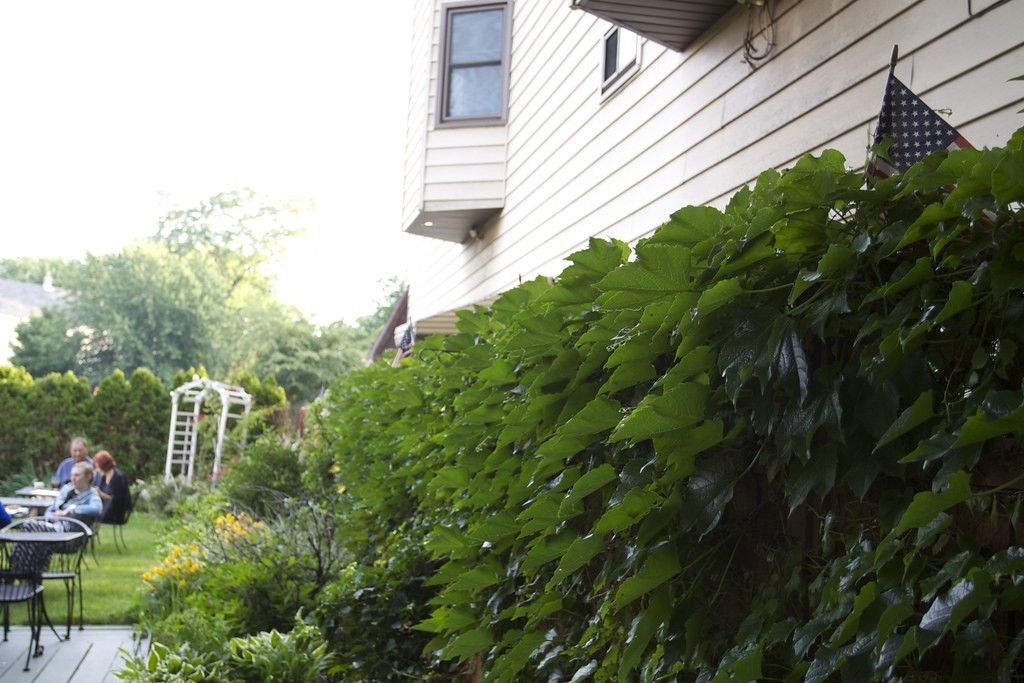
[35, 482, 44, 488]
[51, 477, 60, 490]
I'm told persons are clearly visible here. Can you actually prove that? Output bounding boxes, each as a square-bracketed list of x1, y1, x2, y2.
[93, 450, 131, 523]
[0, 437, 104, 587]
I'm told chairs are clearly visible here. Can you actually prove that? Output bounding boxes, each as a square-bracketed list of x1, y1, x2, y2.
[0, 489, 141, 672]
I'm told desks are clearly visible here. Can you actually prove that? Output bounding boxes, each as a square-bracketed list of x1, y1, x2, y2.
[15, 488, 61, 498]
[0, 531, 84, 657]
[0, 496, 54, 507]
